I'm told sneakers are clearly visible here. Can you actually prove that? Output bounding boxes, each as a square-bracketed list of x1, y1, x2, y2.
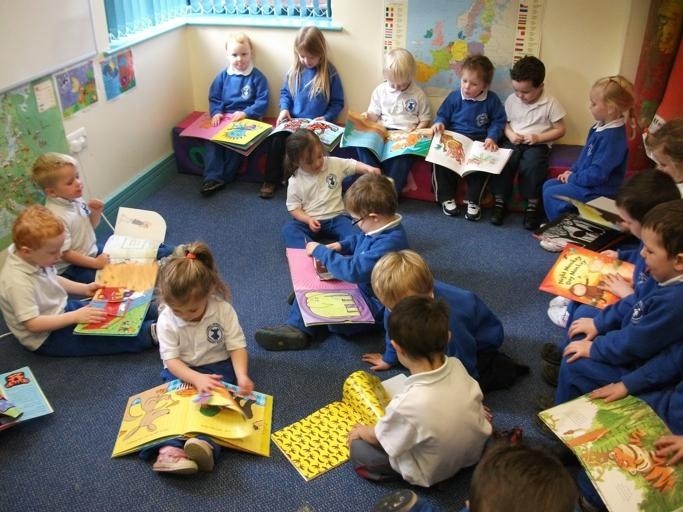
[184, 438, 215, 473]
[255, 325, 307, 351]
[153, 446, 198, 475]
[542, 344, 563, 388]
[200, 179, 223, 193]
[373, 489, 418, 511]
[547, 296, 570, 329]
[260, 182, 275, 199]
[497, 427, 522, 447]
[442, 195, 482, 220]
[524, 206, 565, 252]
[490, 201, 506, 223]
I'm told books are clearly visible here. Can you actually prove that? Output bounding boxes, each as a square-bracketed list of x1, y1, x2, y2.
[269, 370, 410, 482]
[534, 384, 682, 511]
[340, 106, 433, 163]
[110, 376, 273, 458]
[275, 111, 347, 154]
[282, 245, 377, 331]
[0, 367, 54, 434]
[68, 261, 157, 336]
[176, 108, 272, 160]
[100, 205, 167, 261]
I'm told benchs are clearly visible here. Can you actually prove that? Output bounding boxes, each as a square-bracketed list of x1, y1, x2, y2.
[170, 112, 585, 213]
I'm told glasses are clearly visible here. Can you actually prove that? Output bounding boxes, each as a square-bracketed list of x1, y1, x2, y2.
[351, 217, 364, 225]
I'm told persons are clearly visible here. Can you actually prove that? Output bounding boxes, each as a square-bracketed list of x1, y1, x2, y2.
[355, 49, 567, 229]
[534, 73, 683, 438]
[283, 128, 386, 244]
[258, 27, 345, 199]
[31, 150, 185, 285]
[197, 28, 270, 195]
[138, 239, 254, 475]
[461, 440, 590, 510]
[576, 346, 681, 511]
[361, 248, 532, 422]
[254, 173, 409, 350]
[346, 296, 523, 487]
[0, 204, 165, 358]
